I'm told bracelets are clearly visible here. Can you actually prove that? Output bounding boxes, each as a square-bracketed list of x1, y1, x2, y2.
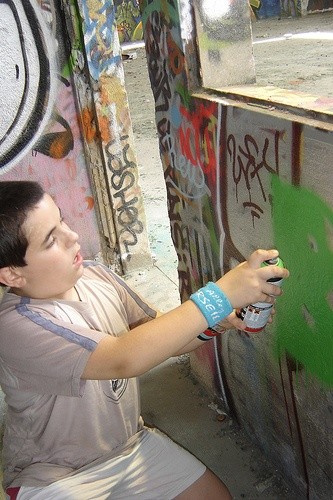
[188, 280, 233, 329]
[0, 180, 290, 500]
[196, 324, 225, 341]
